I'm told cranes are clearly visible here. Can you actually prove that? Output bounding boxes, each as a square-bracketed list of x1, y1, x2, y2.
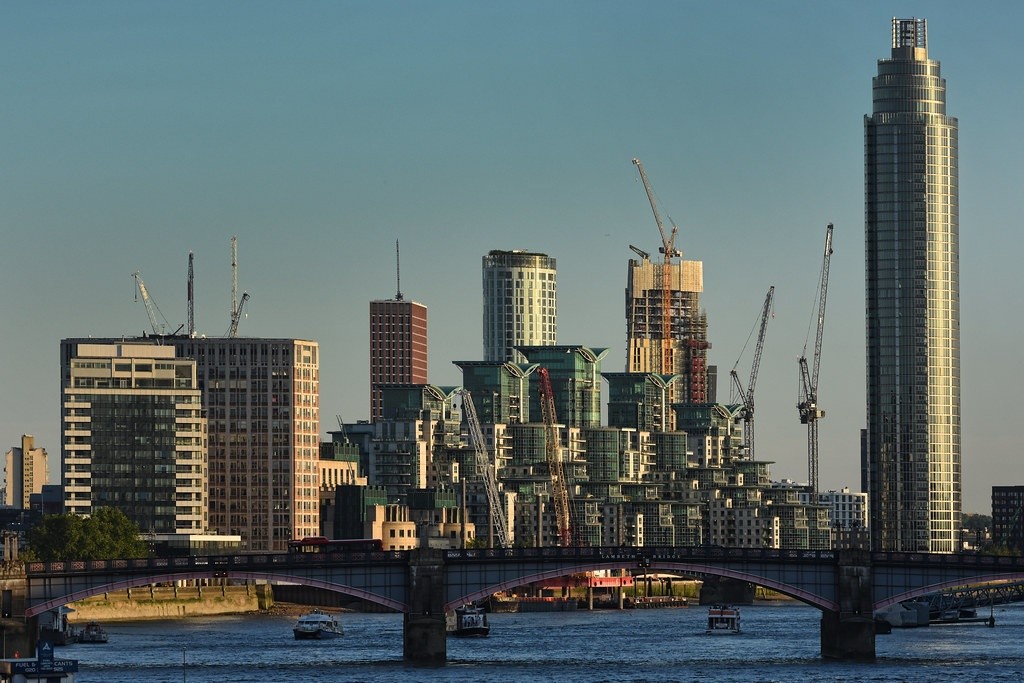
[185, 252, 195, 332]
[131, 270, 166, 333]
[224, 235, 251, 338]
[795, 221, 836, 500]
[729, 285, 775, 459]
[631, 158, 683, 373]
[535, 367, 575, 546]
[460, 389, 513, 549]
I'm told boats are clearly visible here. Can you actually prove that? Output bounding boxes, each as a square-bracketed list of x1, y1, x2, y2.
[705, 602, 742, 634]
[446, 598, 491, 638]
[292, 606, 346, 639]
[77, 621, 109, 643]
